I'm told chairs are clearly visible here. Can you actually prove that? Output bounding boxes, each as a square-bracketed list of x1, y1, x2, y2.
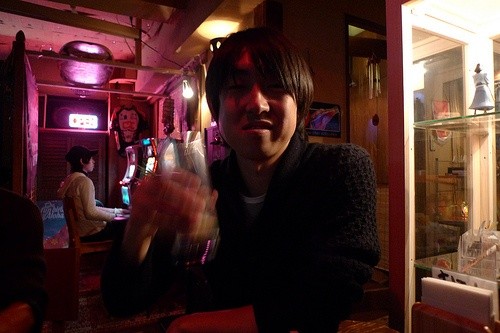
[62, 196, 114, 324]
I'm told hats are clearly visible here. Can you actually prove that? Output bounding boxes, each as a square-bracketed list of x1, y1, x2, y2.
[66, 146, 99, 162]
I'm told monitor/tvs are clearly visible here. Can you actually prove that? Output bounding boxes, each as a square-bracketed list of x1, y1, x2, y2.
[121, 157, 157, 205]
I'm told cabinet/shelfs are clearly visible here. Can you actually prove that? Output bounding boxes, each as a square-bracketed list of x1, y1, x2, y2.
[414, 109, 500, 289]
[432, 157, 468, 255]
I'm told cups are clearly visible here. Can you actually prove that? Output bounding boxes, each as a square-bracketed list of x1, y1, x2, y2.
[157, 130, 219, 245]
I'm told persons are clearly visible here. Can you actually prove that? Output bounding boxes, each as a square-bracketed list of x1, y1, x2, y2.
[102, 28, 382, 333]
[468, 63, 495, 115]
[56, 145, 131, 278]
